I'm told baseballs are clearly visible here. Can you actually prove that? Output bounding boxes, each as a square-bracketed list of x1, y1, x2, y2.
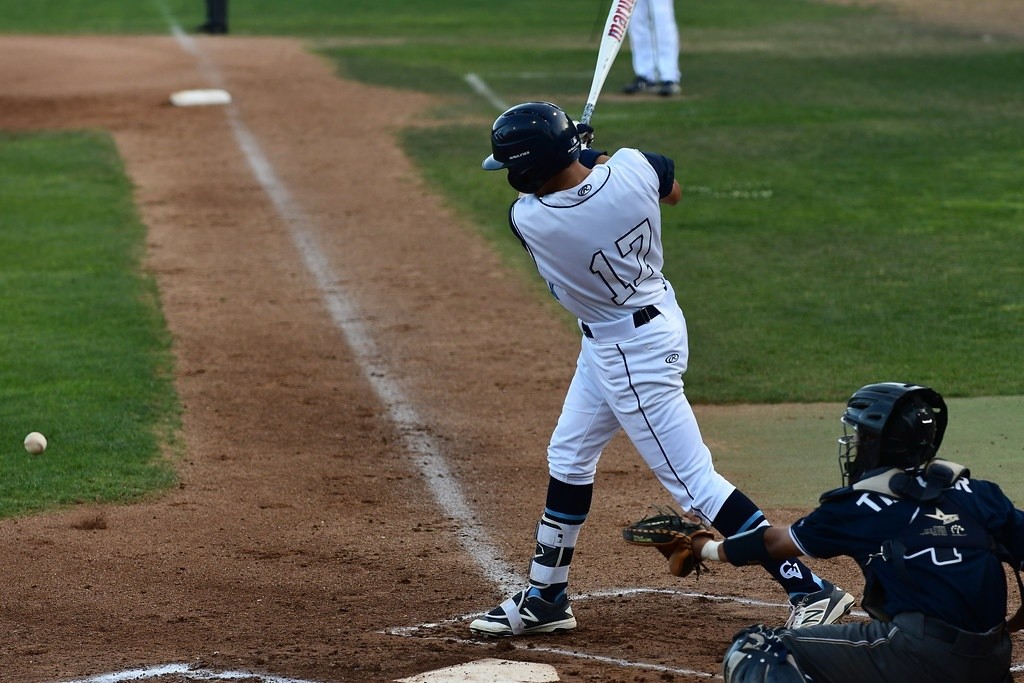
[23, 431, 48, 454]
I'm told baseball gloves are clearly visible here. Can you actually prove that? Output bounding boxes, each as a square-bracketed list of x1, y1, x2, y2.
[621, 513, 714, 578]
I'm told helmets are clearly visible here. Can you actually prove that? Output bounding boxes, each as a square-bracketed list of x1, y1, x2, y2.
[838, 383, 948, 475]
[482, 101, 581, 194]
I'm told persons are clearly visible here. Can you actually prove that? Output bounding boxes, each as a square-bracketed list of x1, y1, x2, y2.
[623, 0, 682, 96]
[196, 0, 229, 33]
[471, 100, 855, 635]
[623, 383, 1024, 683]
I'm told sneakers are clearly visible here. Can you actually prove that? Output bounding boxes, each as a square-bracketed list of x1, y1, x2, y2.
[658, 81, 681, 96]
[469, 586, 577, 638]
[784, 580, 855, 629]
[623, 76, 656, 94]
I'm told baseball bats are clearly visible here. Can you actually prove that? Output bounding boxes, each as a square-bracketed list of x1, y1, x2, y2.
[580, 0, 638, 143]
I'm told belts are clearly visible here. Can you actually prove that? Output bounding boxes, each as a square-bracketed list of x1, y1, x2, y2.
[582, 303, 662, 339]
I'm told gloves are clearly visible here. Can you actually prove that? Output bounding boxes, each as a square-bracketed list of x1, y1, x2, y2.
[573, 119, 594, 147]
[578, 145, 608, 169]
